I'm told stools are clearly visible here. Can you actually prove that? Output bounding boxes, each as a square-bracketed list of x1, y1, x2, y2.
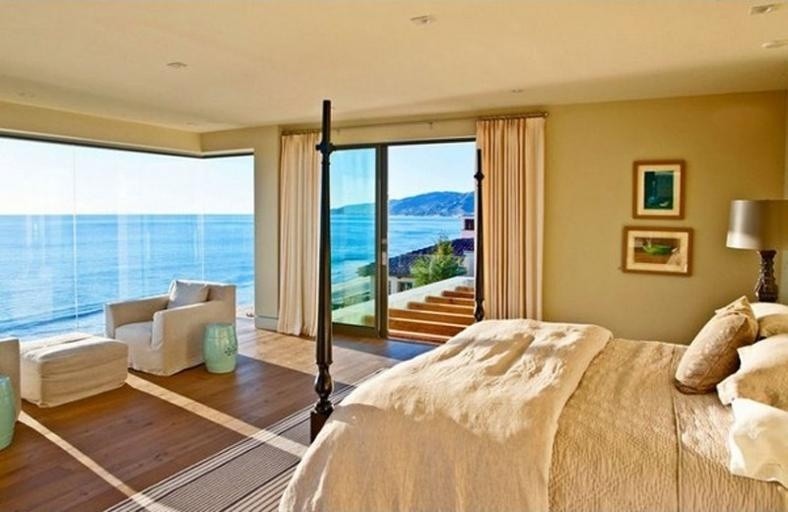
[202, 322, 236, 376]
[0, 375, 17, 451]
[20, 333, 129, 410]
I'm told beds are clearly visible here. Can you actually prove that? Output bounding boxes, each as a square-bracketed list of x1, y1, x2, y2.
[280, 98, 788, 512]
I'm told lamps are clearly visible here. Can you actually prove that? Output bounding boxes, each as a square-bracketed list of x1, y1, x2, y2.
[725, 199, 787, 303]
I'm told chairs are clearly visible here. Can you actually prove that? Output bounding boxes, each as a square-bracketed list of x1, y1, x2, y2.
[102, 279, 236, 378]
[0, 337, 20, 419]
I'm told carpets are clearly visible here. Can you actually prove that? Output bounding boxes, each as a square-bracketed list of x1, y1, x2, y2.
[103, 366, 387, 512]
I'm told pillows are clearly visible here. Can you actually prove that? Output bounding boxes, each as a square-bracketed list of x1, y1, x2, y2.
[677, 294, 787, 490]
[166, 281, 210, 310]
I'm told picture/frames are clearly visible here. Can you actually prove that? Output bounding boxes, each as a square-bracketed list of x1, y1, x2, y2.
[632, 159, 686, 219]
[621, 225, 693, 277]
